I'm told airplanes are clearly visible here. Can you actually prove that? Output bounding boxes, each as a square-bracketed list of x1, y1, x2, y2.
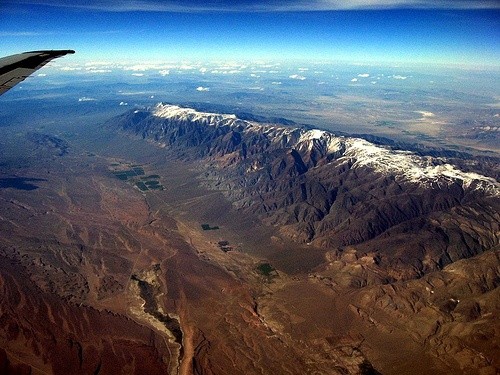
[0, 48, 75, 100]
